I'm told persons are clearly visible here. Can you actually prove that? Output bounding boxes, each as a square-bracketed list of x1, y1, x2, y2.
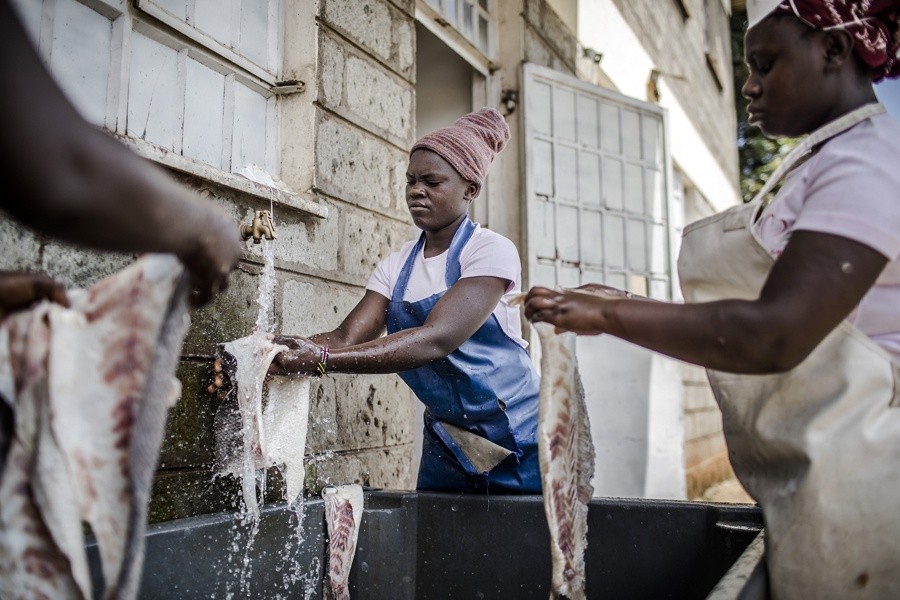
[0, 1, 243, 320]
[523, 1, 900, 600]
[272, 107, 542, 495]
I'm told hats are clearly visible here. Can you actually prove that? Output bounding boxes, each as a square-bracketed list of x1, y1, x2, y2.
[408, 108, 511, 187]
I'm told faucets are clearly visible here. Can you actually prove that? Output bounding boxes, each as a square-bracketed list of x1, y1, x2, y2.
[251, 210, 279, 244]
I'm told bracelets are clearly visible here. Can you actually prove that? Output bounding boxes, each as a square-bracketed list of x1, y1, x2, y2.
[314, 347, 329, 379]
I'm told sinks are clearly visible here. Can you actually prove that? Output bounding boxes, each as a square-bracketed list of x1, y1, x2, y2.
[2, 484, 771, 600]
